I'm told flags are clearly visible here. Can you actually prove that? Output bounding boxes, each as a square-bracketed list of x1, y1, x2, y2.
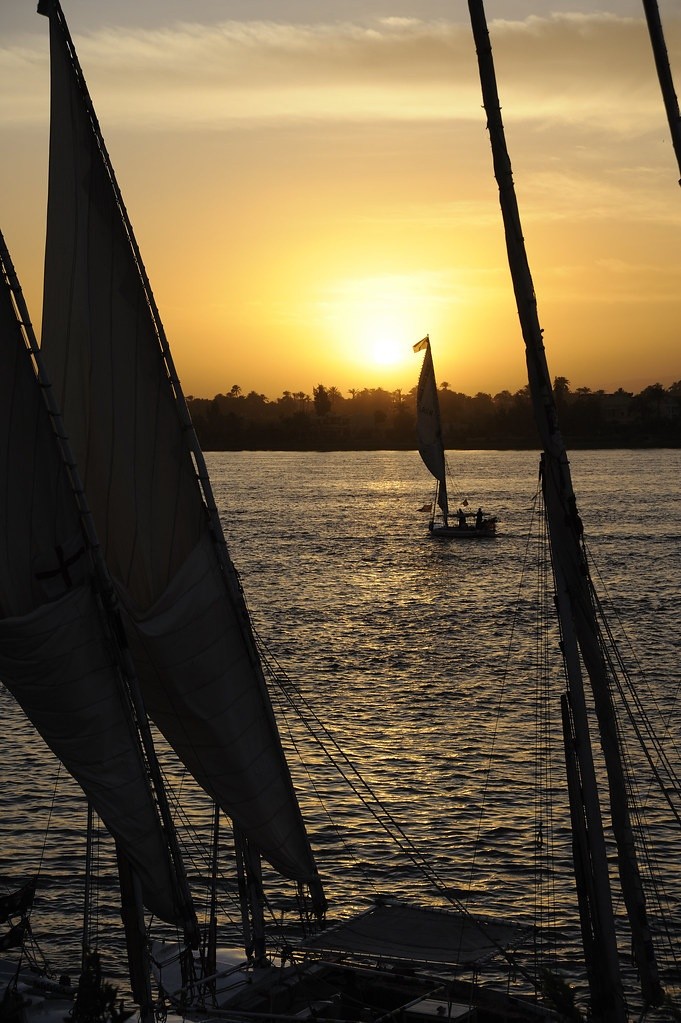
[414, 338, 428, 352]
[418, 505, 432, 512]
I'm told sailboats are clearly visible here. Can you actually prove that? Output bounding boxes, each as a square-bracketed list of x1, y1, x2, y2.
[414, 334, 500, 537]
[0, 0, 681, 1023]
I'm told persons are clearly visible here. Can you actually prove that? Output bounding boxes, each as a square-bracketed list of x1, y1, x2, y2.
[457, 509, 466, 525]
[476, 508, 482, 523]
